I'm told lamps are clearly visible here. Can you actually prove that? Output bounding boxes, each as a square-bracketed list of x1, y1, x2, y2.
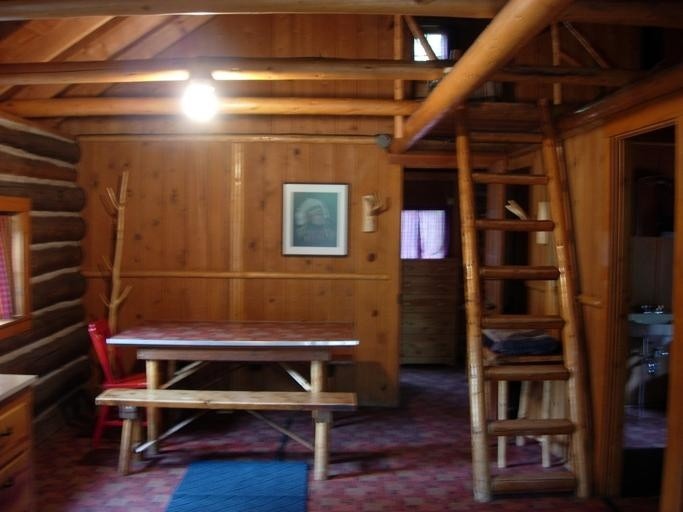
[178, 72, 223, 125]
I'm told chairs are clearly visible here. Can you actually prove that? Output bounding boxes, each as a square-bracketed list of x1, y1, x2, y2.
[85, 316, 161, 448]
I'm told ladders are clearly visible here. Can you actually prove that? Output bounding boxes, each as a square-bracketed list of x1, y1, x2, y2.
[453, 99, 591, 505]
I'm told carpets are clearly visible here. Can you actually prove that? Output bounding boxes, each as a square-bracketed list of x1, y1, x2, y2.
[166, 460, 309, 512]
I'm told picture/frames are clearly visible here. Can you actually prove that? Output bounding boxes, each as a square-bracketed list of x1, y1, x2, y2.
[281, 182, 349, 257]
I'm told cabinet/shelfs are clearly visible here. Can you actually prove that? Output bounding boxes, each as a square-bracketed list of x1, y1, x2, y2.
[0, 372, 40, 512]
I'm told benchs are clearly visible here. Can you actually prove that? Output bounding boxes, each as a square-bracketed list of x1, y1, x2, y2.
[91, 387, 359, 481]
